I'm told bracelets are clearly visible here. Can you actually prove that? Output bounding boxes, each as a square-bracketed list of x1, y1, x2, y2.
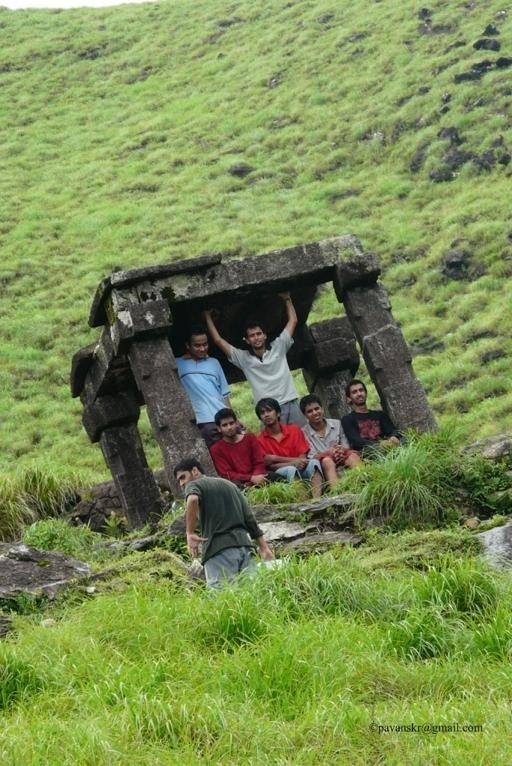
[284, 297, 292, 302]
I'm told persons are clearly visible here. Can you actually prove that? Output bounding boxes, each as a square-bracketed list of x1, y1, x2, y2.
[202, 291, 307, 430]
[254, 397, 323, 501]
[208, 408, 288, 492]
[299, 395, 363, 484]
[174, 458, 274, 595]
[174, 326, 245, 448]
[342, 380, 409, 464]
